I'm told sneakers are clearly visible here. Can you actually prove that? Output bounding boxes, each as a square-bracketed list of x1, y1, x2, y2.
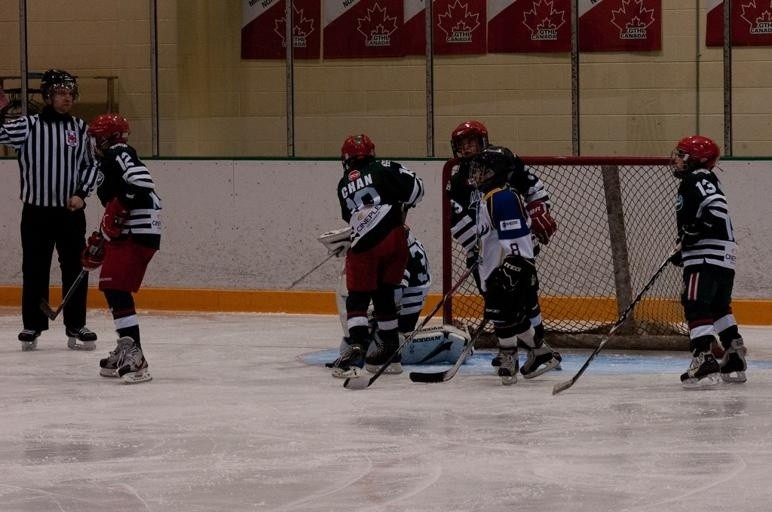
[65, 324, 97, 341]
[17, 328, 42, 342]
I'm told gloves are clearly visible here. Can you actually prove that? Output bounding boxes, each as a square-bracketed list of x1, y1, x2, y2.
[80, 231, 105, 272]
[678, 220, 702, 245]
[102, 197, 130, 243]
[526, 198, 557, 245]
[672, 251, 684, 267]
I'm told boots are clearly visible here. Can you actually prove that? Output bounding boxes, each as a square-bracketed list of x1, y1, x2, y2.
[720, 333, 747, 373]
[492, 344, 519, 376]
[100, 336, 148, 377]
[365, 330, 402, 365]
[680, 339, 721, 381]
[519, 324, 554, 375]
[334, 335, 370, 369]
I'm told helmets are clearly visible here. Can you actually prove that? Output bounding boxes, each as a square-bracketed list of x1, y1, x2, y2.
[341, 135, 376, 168]
[86, 112, 130, 141]
[41, 69, 78, 102]
[451, 121, 489, 161]
[670, 135, 720, 177]
[474, 146, 516, 193]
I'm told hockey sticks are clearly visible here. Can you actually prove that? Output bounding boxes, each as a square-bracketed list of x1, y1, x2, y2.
[344, 260, 485, 389]
[553, 242, 682, 395]
[40, 236, 106, 321]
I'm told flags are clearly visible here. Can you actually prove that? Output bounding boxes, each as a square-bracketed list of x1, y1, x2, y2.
[705, 1, 772, 47]
[404, 1, 486, 58]
[322, 1, 407, 60]
[576, 1, 662, 53]
[239, 1, 321, 60]
[486, 1, 573, 54]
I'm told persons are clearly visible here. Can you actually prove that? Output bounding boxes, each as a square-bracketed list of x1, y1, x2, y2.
[470, 147, 557, 377]
[333, 132, 426, 372]
[667, 135, 748, 382]
[0, 67, 99, 352]
[334, 225, 475, 367]
[80, 110, 163, 386]
[444, 119, 555, 369]
